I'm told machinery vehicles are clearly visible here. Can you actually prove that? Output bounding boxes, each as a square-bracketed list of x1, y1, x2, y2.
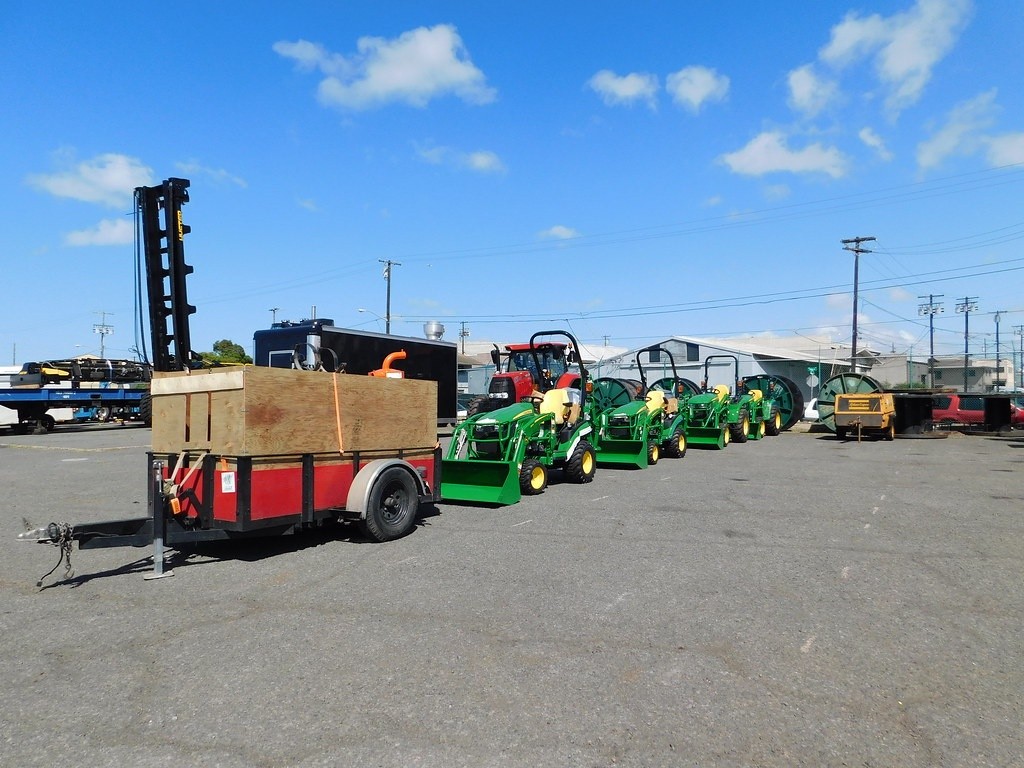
[428, 333, 785, 504]
